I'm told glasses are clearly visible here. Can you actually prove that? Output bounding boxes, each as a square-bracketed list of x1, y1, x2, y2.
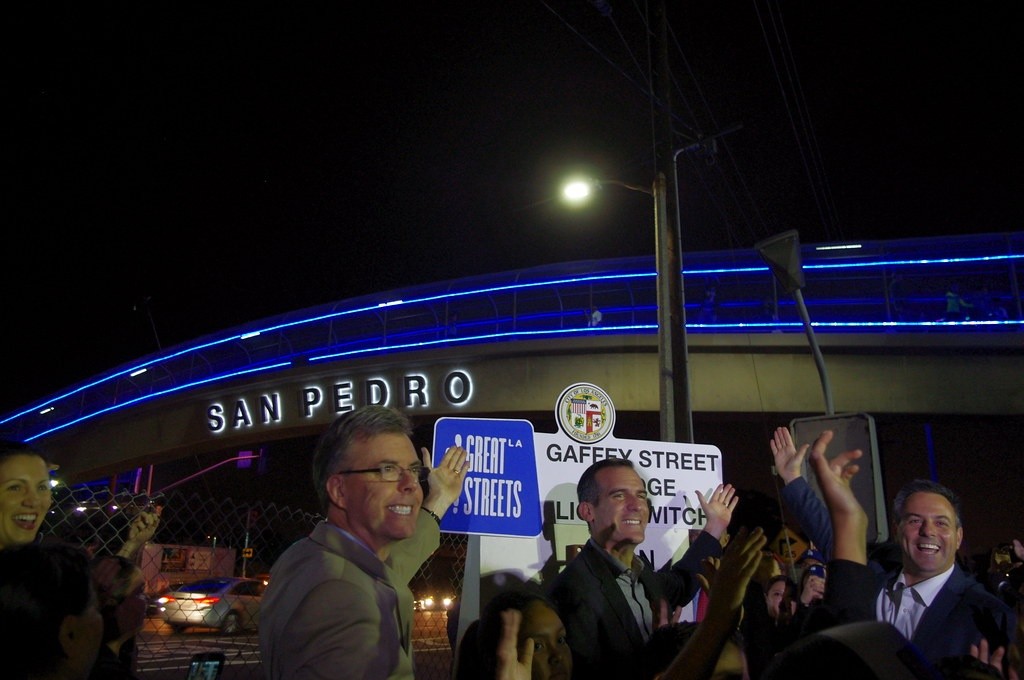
[338, 460, 428, 482]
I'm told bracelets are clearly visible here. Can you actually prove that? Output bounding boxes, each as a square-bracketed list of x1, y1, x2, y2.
[421, 507, 441, 525]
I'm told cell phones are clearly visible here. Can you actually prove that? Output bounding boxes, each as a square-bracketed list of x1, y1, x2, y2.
[185, 652, 225, 680]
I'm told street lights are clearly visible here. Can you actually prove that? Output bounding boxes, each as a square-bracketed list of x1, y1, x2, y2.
[556, 170, 678, 446]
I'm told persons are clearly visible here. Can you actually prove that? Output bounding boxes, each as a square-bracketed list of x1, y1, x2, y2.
[458, 593, 572, 680]
[652, 426, 1024, 680]
[260, 407, 470, 680]
[550, 459, 738, 680]
[0, 442, 160, 680]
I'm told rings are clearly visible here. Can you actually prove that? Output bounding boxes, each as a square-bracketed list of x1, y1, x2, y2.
[722, 502, 728, 507]
[453, 469, 459, 474]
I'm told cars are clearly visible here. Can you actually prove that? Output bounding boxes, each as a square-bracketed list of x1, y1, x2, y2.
[152, 576, 266, 637]
[410, 585, 458, 613]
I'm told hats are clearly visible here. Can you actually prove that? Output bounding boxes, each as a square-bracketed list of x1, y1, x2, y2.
[795, 547, 824, 567]
[801, 564, 827, 579]
